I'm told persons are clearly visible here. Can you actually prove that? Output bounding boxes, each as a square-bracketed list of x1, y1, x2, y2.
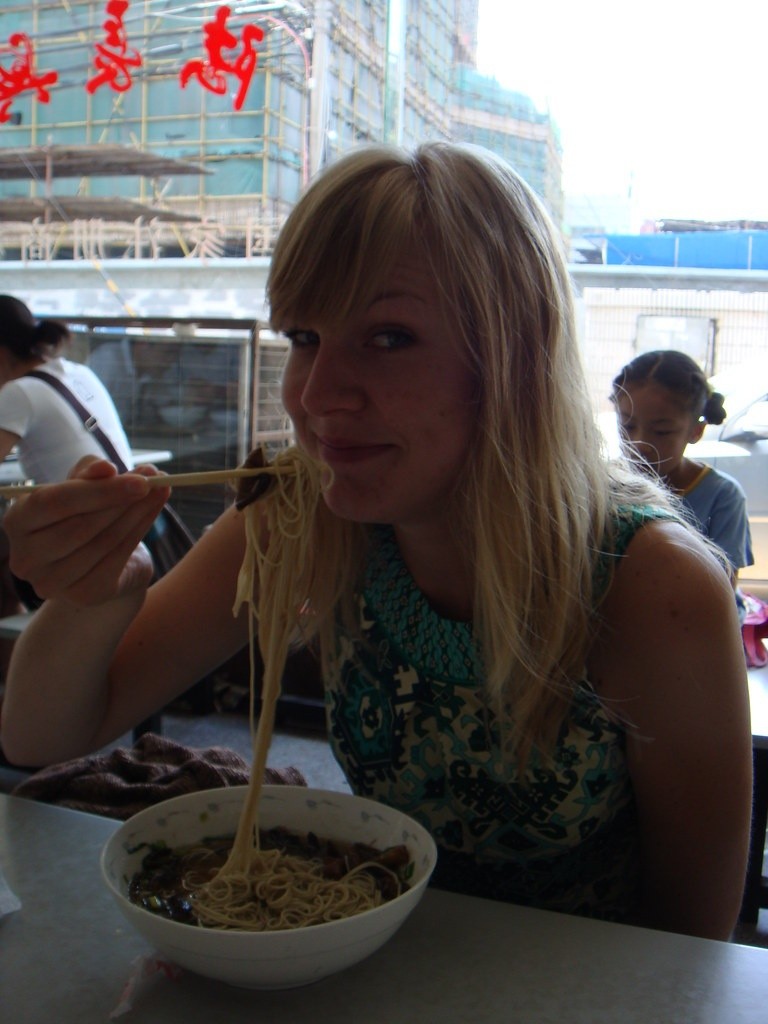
[81, 340, 213, 427]
[609, 350, 756, 585]
[0, 294, 134, 613]
[0, 141, 752, 940]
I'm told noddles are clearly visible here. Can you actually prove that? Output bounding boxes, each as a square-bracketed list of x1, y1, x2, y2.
[129, 435, 410, 932]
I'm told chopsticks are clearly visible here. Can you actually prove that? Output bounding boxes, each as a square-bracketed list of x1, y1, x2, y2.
[0, 462, 304, 499]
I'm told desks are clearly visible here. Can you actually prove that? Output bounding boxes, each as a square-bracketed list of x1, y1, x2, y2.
[1, 791, 768, 1024]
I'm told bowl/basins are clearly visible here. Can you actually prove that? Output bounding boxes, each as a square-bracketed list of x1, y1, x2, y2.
[100, 785, 437, 989]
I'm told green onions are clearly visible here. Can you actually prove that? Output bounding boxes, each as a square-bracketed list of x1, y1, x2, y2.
[132, 831, 356, 925]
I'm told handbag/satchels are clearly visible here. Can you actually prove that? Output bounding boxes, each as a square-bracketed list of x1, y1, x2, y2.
[740, 591, 768, 668]
[146, 503, 195, 575]
